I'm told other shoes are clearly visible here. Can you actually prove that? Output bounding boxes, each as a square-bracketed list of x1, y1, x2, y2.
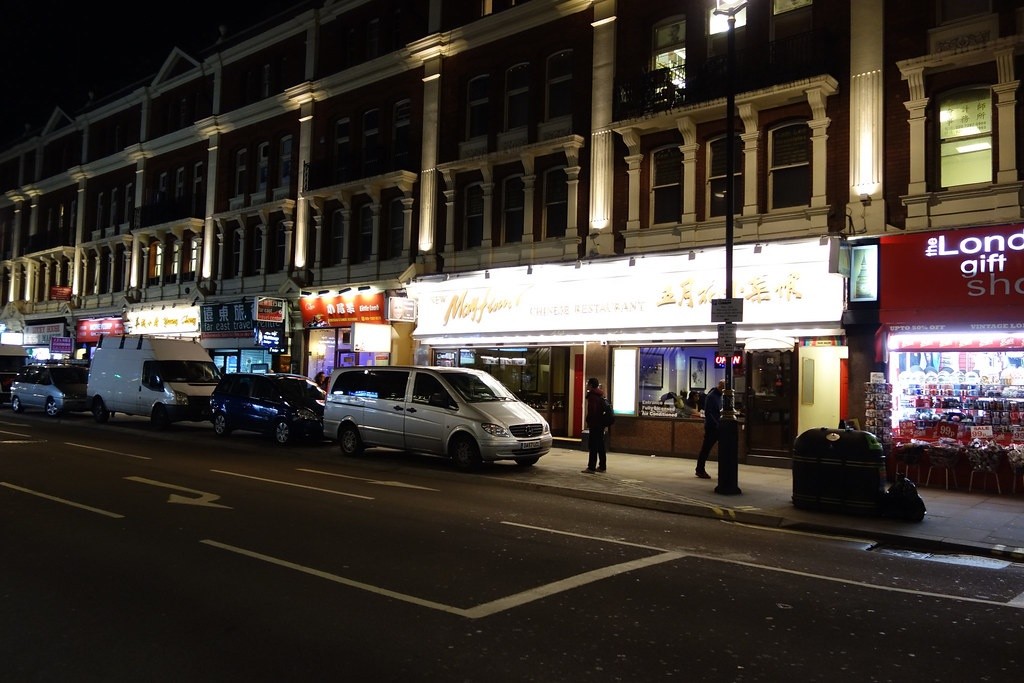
[695, 471, 711, 478]
[595, 467, 606, 473]
[581, 469, 595, 474]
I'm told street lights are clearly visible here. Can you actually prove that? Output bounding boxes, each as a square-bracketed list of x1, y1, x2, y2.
[708, 0, 749, 501]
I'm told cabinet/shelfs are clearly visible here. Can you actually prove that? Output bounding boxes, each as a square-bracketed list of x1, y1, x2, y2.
[898, 383, 1024, 428]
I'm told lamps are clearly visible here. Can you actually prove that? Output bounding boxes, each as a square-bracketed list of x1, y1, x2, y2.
[593, 228, 601, 237]
[860, 194, 870, 202]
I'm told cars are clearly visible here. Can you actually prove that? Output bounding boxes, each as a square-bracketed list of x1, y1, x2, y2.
[207, 369, 326, 446]
[8, 363, 89, 419]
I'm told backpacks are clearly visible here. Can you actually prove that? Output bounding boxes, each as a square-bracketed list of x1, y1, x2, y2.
[591, 392, 615, 427]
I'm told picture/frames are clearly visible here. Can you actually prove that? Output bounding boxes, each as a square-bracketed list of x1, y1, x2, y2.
[689, 356, 706, 390]
[640, 352, 664, 388]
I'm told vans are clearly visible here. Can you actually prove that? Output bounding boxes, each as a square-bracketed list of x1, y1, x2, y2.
[83, 331, 223, 430]
[323, 363, 552, 469]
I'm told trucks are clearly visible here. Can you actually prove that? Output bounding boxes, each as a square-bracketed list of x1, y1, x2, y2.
[0, 344, 30, 403]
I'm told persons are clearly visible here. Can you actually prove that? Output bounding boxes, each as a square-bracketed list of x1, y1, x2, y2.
[695, 379, 725, 478]
[582, 378, 614, 474]
[687, 391, 701, 411]
[315, 372, 331, 391]
